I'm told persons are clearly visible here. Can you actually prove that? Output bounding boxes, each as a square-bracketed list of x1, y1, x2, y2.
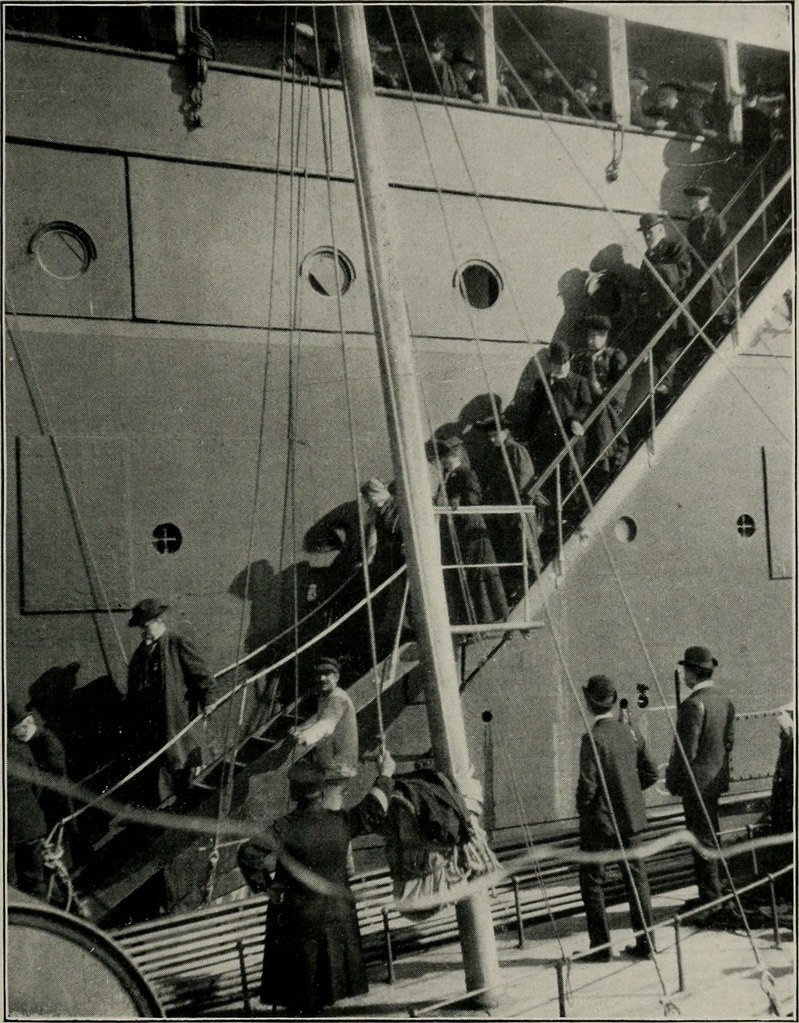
[663, 645, 736, 920]
[683, 181, 733, 328]
[307, 479, 415, 672]
[273, 20, 342, 80]
[123, 599, 218, 813]
[6, 737, 66, 910]
[626, 65, 792, 229]
[366, 32, 611, 121]
[571, 675, 659, 960]
[286, 653, 360, 876]
[430, 313, 631, 629]
[236, 749, 396, 1012]
[7, 701, 79, 871]
[749, 702, 793, 916]
[634, 210, 693, 394]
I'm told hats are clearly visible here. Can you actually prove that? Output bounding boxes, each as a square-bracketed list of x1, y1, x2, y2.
[657, 78, 687, 91]
[629, 66, 650, 83]
[678, 646, 717, 670]
[313, 657, 341, 672]
[683, 186, 711, 199]
[452, 48, 477, 68]
[583, 314, 611, 329]
[636, 212, 666, 232]
[544, 340, 574, 364]
[7, 703, 36, 730]
[287, 760, 325, 799]
[578, 65, 598, 83]
[479, 415, 512, 430]
[582, 674, 616, 708]
[437, 436, 464, 455]
[127, 597, 168, 628]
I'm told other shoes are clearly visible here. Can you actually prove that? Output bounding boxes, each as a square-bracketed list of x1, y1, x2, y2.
[625, 944, 653, 959]
[572, 951, 612, 964]
